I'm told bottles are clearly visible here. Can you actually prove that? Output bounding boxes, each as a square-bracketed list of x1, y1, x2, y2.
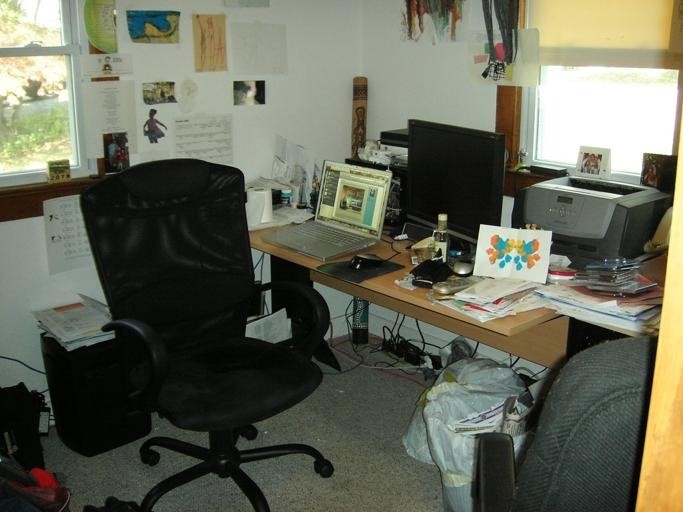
[434, 213, 448, 264]
[281, 188, 292, 206]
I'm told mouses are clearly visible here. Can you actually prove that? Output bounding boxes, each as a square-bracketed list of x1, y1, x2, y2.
[453, 259, 474, 276]
[350, 254, 384, 271]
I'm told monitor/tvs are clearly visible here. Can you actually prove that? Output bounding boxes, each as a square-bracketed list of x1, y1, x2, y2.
[404, 119, 505, 245]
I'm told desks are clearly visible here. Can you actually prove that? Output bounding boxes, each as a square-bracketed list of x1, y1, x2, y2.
[247, 211, 669, 379]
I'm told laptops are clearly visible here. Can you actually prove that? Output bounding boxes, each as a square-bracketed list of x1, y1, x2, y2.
[261, 160, 393, 262]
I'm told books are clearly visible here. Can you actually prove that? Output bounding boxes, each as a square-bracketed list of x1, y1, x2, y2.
[35, 322, 119, 354]
[30, 295, 112, 341]
[281, 186, 292, 206]
[429, 256, 664, 335]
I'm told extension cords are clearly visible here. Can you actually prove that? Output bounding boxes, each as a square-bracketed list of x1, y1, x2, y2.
[400, 340, 441, 370]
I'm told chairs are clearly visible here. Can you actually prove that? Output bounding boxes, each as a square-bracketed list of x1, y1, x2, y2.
[462, 331, 656, 512]
[76, 155, 339, 511]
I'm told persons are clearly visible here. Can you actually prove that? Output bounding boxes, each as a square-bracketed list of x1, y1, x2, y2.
[585, 153, 600, 174]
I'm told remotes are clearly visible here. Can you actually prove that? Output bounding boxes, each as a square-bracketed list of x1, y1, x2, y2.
[432, 279, 475, 294]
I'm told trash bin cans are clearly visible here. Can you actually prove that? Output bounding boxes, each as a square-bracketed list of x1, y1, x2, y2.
[423, 394, 536, 512]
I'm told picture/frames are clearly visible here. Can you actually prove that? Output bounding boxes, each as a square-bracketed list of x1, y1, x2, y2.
[572, 143, 613, 181]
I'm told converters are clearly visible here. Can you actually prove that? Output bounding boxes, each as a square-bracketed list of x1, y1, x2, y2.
[404, 353, 421, 366]
[530, 164, 567, 178]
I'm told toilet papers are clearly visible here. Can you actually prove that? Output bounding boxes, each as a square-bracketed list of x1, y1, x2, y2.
[245, 187, 272, 224]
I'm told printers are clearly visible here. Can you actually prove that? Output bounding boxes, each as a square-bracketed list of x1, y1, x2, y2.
[513, 175, 672, 269]
